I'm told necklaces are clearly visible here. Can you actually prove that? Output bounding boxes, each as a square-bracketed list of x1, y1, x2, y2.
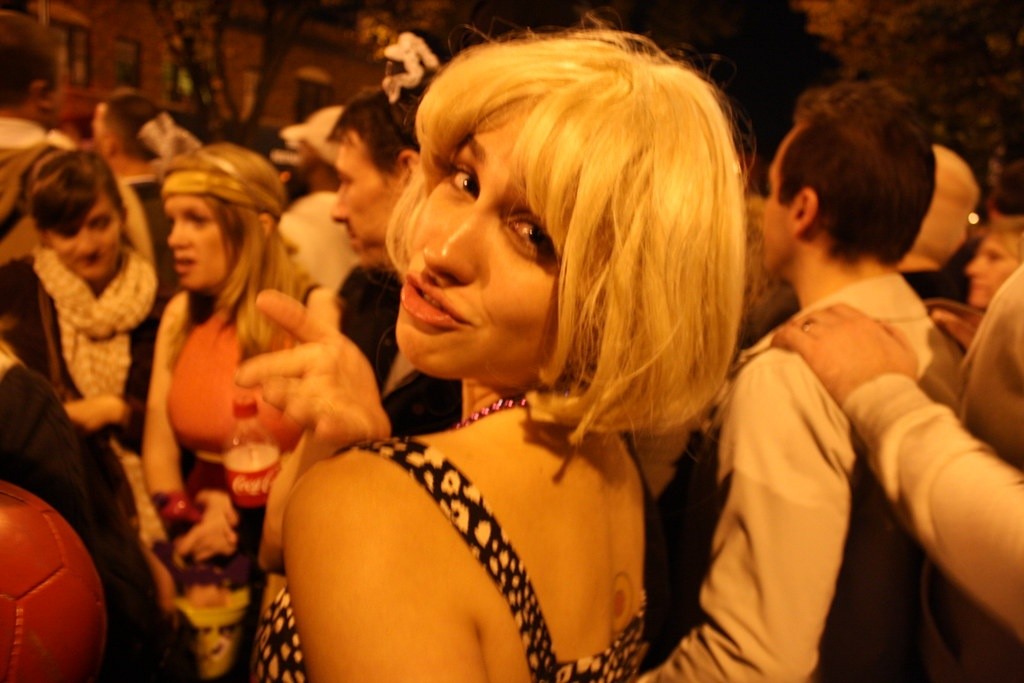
[451, 394, 529, 429]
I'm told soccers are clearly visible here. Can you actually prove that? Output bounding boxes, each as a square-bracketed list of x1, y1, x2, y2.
[1, 477, 110, 683]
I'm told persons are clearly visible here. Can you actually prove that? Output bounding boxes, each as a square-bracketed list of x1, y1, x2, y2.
[0, 38, 440, 683]
[237, 26, 746, 682]
[636, 77, 1024, 682]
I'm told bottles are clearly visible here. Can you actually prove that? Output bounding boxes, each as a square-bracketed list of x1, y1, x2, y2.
[223, 394, 280, 556]
[152, 487, 250, 594]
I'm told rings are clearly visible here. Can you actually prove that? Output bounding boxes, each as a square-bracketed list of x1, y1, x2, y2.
[802, 318, 815, 333]
